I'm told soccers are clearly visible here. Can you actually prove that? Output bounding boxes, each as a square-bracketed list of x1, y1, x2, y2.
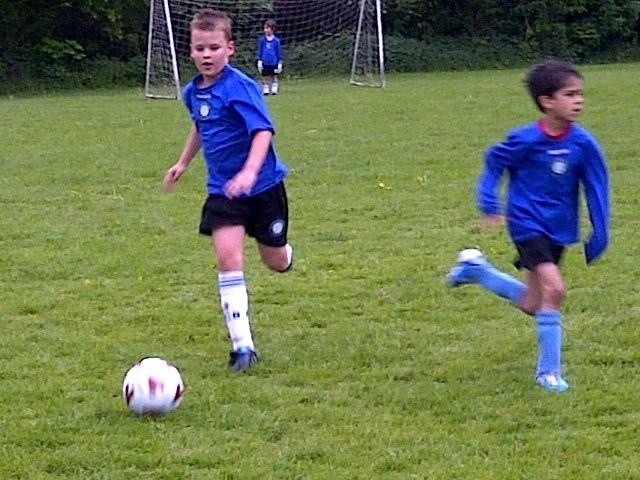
[123, 357, 185, 416]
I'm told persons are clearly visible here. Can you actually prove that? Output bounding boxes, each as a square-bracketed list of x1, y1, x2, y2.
[162, 10, 293, 372]
[445, 60, 611, 395]
[253, 18, 283, 95]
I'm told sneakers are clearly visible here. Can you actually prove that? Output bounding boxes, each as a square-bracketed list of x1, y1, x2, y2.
[271, 85, 278, 95]
[262, 87, 269, 95]
[536, 372, 569, 392]
[226, 347, 260, 373]
[445, 248, 483, 288]
